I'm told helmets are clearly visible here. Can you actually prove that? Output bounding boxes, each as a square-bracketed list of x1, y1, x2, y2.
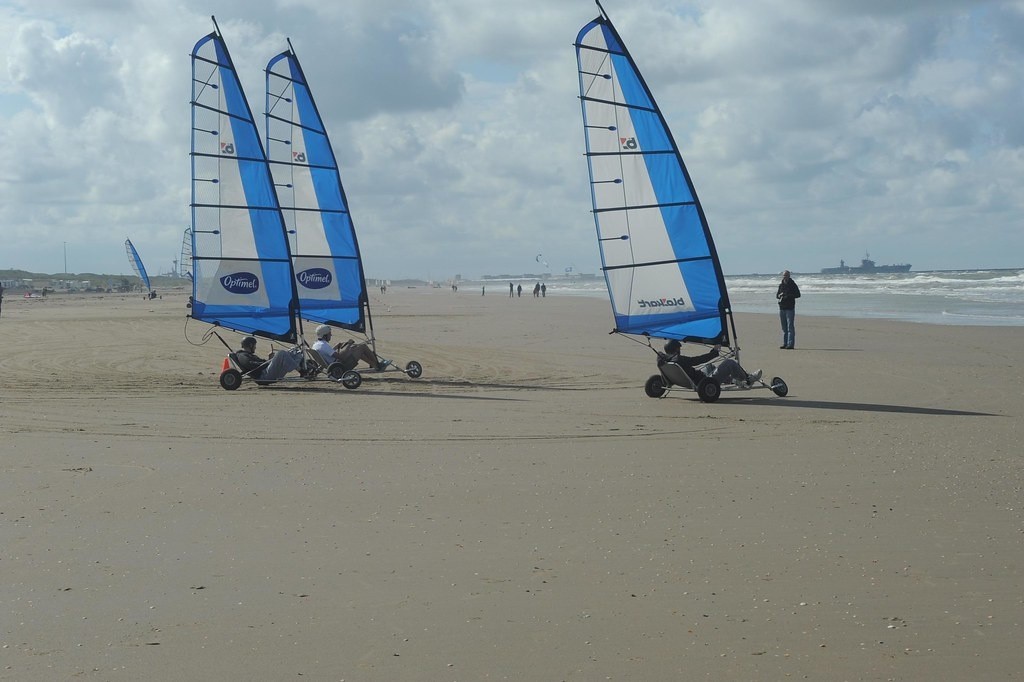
[316, 324, 331, 338]
[663, 339, 682, 354]
[240, 337, 256, 348]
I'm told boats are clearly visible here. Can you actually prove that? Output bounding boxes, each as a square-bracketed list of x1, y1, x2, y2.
[821, 249, 912, 274]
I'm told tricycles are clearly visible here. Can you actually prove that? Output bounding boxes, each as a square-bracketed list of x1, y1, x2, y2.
[125, 238, 163, 301]
[576, 0, 789, 401]
[186, 15, 423, 388]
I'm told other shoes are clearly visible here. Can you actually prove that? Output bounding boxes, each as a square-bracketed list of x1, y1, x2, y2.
[780, 345, 787, 349]
[300, 362, 315, 378]
[785, 346, 794, 349]
[376, 359, 392, 372]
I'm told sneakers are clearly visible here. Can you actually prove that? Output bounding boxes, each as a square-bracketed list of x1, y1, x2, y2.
[744, 369, 762, 388]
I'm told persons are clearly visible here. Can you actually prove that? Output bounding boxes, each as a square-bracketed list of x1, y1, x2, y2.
[381, 285, 386, 294]
[42, 286, 47, 297]
[775, 271, 801, 350]
[517, 284, 522, 297]
[541, 283, 546, 297]
[533, 282, 540, 298]
[237, 336, 313, 384]
[312, 324, 388, 372]
[148, 289, 157, 300]
[452, 284, 457, 292]
[656, 339, 763, 387]
[510, 283, 513, 297]
